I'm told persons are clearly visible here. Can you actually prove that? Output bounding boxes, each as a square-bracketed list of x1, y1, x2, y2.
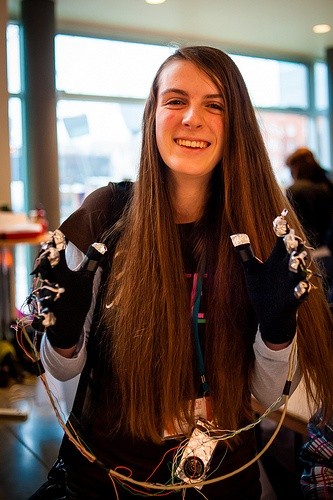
[27, 46, 333, 500]
[283, 147, 333, 285]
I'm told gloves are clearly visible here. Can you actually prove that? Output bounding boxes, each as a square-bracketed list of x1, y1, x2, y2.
[228, 210, 321, 342]
[11, 231, 108, 348]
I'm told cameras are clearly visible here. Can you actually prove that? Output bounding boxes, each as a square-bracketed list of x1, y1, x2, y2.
[173, 416, 220, 491]
